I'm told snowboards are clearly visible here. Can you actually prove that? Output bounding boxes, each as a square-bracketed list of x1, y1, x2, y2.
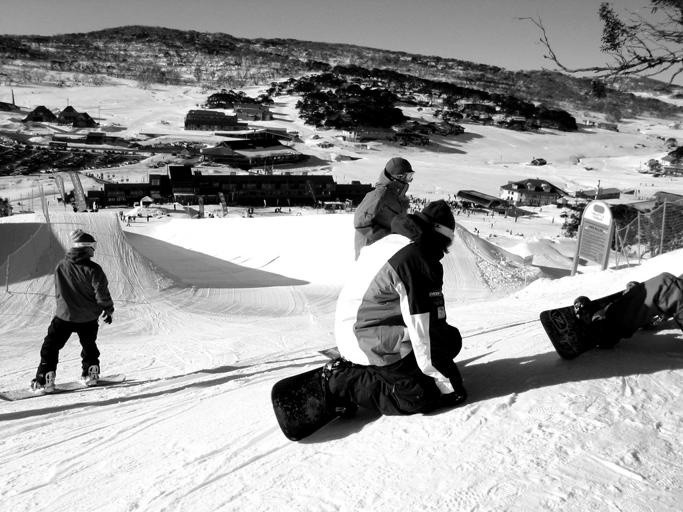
[541, 273, 683, 360]
[271, 326, 462, 442]
[0, 374, 126, 400]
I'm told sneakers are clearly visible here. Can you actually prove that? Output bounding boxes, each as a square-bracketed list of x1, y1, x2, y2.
[31, 378, 55, 396]
[81, 375, 99, 386]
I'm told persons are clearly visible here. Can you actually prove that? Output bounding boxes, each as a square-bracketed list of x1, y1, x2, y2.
[575, 272, 683, 345]
[329, 199, 468, 417]
[354, 158, 415, 261]
[30, 228, 114, 388]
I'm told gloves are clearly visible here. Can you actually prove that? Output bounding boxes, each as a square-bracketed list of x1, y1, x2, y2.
[102, 306, 114, 324]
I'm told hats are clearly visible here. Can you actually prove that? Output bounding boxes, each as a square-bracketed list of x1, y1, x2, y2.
[385, 157, 414, 175]
[421, 199, 454, 231]
[70, 228, 96, 250]
